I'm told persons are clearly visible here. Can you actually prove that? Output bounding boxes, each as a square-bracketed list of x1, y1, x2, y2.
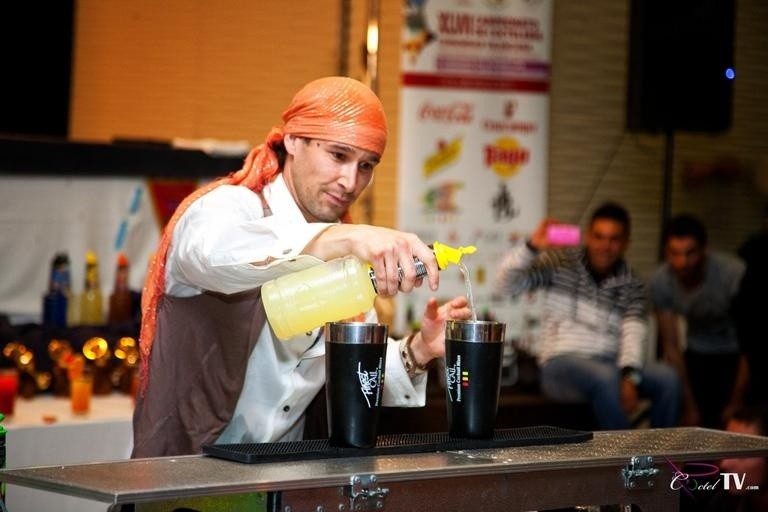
[501, 200, 681, 433]
[130, 74, 473, 456]
[640, 212, 760, 434]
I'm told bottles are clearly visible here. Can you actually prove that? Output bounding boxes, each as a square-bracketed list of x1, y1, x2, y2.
[108, 253, 134, 328]
[260, 243, 478, 342]
[43, 251, 74, 327]
[78, 250, 104, 327]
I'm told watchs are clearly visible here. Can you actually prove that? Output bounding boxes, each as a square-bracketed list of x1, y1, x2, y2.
[620, 365, 643, 385]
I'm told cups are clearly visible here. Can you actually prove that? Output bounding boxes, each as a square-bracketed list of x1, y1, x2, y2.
[0, 367, 18, 425]
[68, 367, 96, 419]
[445, 318, 507, 439]
[323, 321, 390, 448]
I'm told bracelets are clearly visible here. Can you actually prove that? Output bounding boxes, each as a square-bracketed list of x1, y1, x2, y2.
[400, 331, 431, 378]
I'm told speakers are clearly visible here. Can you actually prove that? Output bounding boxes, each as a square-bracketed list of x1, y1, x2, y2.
[626, 0, 738, 136]
